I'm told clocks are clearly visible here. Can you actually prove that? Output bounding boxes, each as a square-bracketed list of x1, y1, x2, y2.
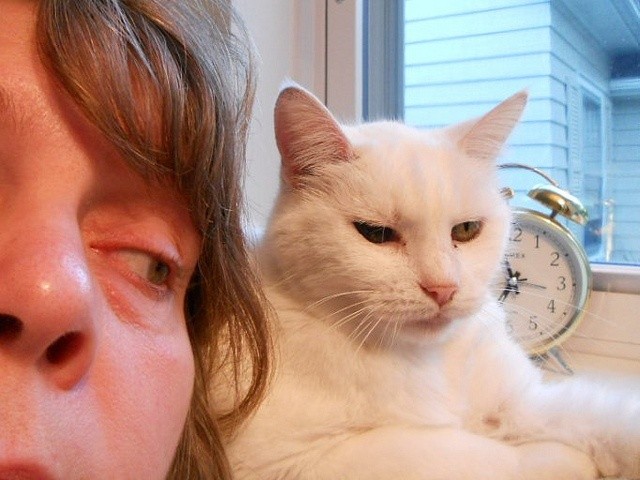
[493, 163, 594, 377]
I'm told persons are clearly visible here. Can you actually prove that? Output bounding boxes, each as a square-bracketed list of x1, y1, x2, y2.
[0, 1, 281, 480]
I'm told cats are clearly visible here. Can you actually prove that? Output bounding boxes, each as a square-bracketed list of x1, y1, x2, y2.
[201, 84, 640, 480]
